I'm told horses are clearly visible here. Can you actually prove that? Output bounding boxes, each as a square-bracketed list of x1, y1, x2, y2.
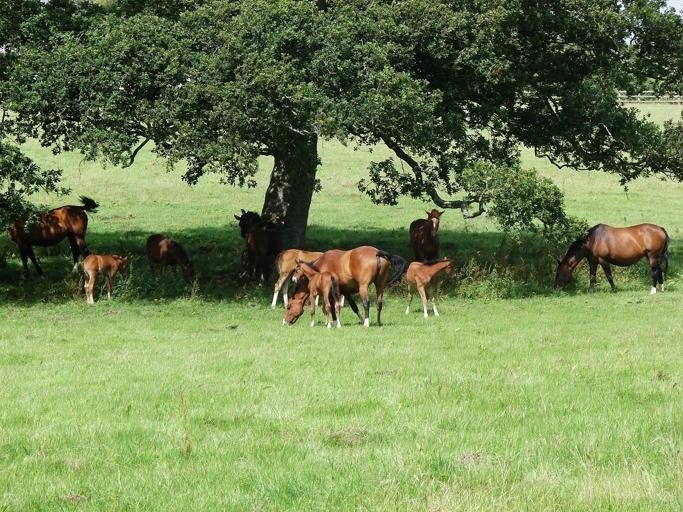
[268, 245, 457, 328]
[0, 194, 100, 278]
[80, 254, 128, 305]
[408, 208, 444, 265]
[553, 223, 670, 295]
[146, 234, 195, 279]
[234, 208, 282, 288]
